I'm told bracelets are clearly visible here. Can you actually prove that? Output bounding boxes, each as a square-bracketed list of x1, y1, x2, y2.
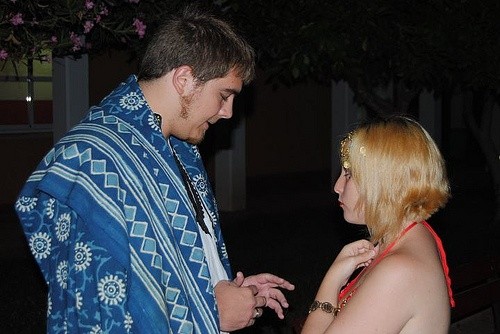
[309, 300, 340, 317]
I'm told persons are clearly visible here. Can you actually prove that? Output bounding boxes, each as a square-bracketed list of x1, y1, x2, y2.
[15, 8, 294, 334]
[301, 116, 455, 334]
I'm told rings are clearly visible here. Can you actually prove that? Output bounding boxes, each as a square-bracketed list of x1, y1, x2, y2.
[255, 308, 259, 318]
